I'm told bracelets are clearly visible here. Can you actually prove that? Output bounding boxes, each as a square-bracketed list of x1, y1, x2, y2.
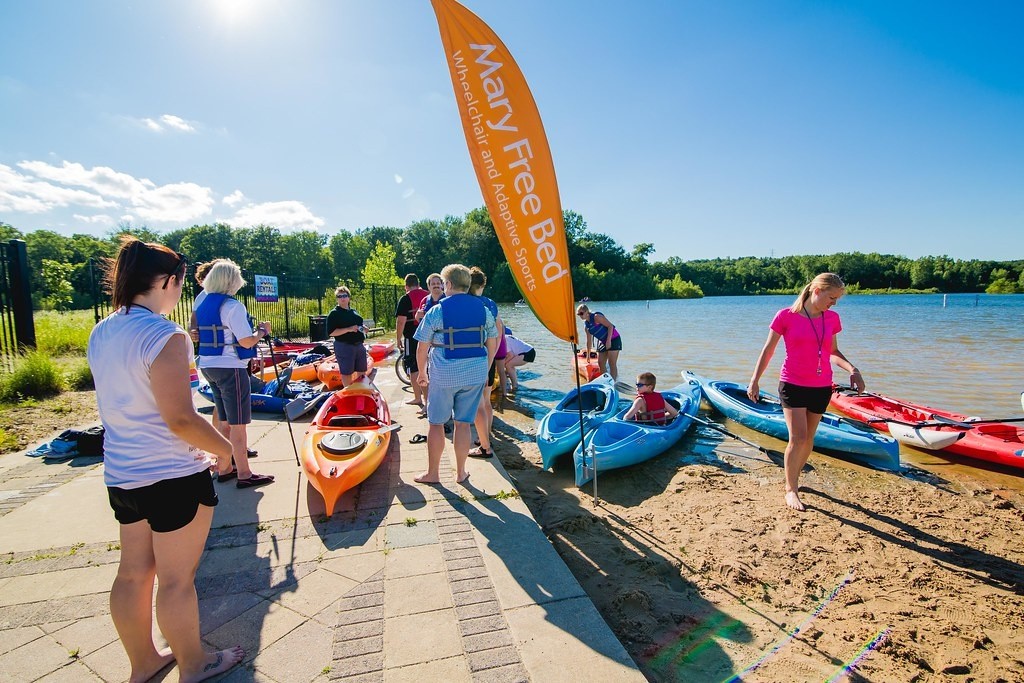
[258, 327, 268, 337]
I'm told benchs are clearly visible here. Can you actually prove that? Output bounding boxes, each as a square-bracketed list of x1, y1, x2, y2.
[364, 319, 385, 338]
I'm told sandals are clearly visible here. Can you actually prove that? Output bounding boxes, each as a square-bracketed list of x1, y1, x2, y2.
[468, 441, 493, 458]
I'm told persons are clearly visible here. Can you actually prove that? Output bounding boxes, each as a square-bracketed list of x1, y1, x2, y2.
[747, 273, 865, 511]
[623, 372, 678, 426]
[576, 304, 622, 382]
[326, 286, 369, 388]
[187, 258, 274, 489]
[414, 263, 498, 482]
[87, 236, 247, 683]
[395, 273, 430, 405]
[415, 267, 536, 458]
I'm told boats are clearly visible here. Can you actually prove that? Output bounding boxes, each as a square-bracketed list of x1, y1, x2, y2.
[491, 368, 500, 392]
[829, 381, 1024, 469]
[197, 342, 396, 413]
[680, 370, 908, 472]
[535, 372, 702, 488]
[302, 374, 392, 518]
[578, 297, 594, 303]
[514, 300, 530, 308]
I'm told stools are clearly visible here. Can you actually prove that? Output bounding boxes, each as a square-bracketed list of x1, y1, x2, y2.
[329, 415, 369, 427]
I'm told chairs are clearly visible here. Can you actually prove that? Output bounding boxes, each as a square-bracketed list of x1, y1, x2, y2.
[636, 409, 666, 427]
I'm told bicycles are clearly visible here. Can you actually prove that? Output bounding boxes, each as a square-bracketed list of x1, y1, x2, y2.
[395, 348, 412, 384]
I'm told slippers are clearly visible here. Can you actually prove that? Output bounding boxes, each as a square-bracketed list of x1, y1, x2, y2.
[409, 434, 427, 444]
[444, 424, 451, 433]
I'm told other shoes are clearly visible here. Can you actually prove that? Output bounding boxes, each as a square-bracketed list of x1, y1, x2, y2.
[217, 467, 236, 482]
[232, 450, 258, 465]
[236, 474, 274, 489]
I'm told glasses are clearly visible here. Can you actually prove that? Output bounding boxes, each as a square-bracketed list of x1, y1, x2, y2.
[636, 383, 651, 388]
[577, 310, 586, 316]
[162, 252, 187, 289]
[336, 294, 349, 298]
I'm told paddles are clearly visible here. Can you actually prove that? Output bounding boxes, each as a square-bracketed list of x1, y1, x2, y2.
[364, 412, 401, 434]
[917, 418, 1024, 427]
[821, 414, 880, 435]
[833, 380, 974, 429]
[614, 381, 815, 471]
